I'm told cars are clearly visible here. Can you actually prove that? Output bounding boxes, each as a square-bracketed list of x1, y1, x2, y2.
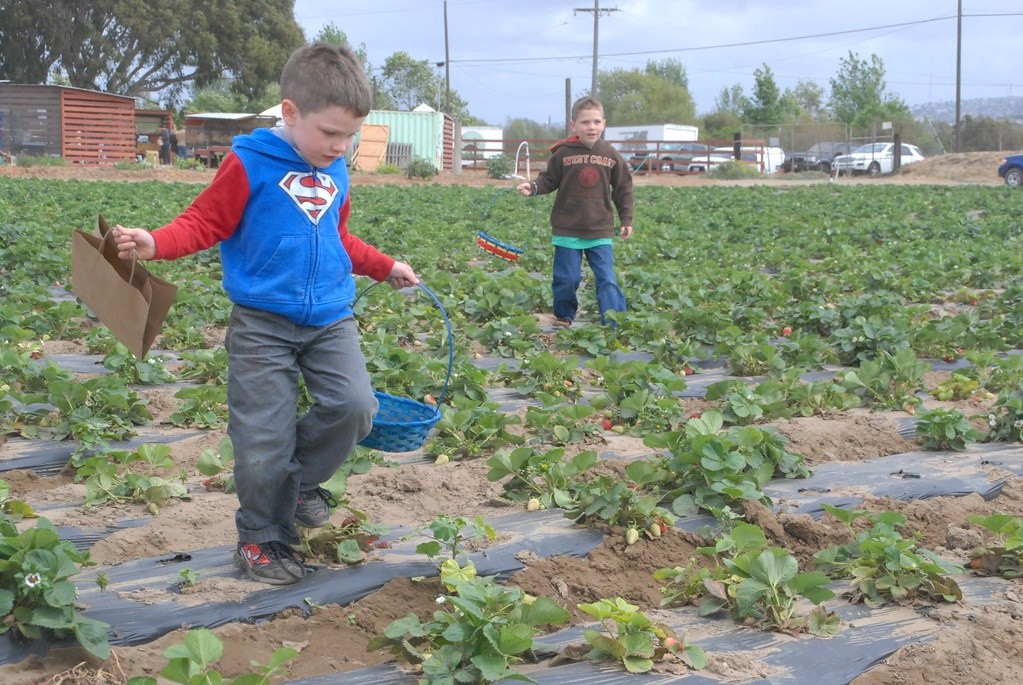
[999, 152, 1023, 185]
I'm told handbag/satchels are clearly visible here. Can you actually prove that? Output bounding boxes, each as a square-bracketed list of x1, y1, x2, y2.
[72, 214, 178, 363]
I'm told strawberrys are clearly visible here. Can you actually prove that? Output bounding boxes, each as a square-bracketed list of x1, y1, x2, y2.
[0, 325, 995, 653]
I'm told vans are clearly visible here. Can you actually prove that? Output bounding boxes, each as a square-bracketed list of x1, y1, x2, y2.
[687, 147, 785, 176]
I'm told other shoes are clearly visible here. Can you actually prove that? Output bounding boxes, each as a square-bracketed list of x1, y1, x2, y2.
[551, 319, 572, 330]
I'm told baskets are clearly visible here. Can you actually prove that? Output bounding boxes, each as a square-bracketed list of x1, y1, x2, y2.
[351, 275, 453, 453]
[477, 188, 536, 264]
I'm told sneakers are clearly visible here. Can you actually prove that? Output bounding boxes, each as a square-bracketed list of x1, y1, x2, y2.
[233, 539, 307, 585]
[294, 487, 338, 528]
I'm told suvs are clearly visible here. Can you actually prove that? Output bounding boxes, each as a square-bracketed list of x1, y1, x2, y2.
[832, 142, 926, 176]
[630, 140, 708, 174]
[782, 140, 862, 173]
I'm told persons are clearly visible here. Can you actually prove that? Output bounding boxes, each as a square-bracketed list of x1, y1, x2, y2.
[517, 97, 634, 329]
[113, 41, 419, 583]
[170, 131, 186, 160]
[159, 124, 170, 165]
